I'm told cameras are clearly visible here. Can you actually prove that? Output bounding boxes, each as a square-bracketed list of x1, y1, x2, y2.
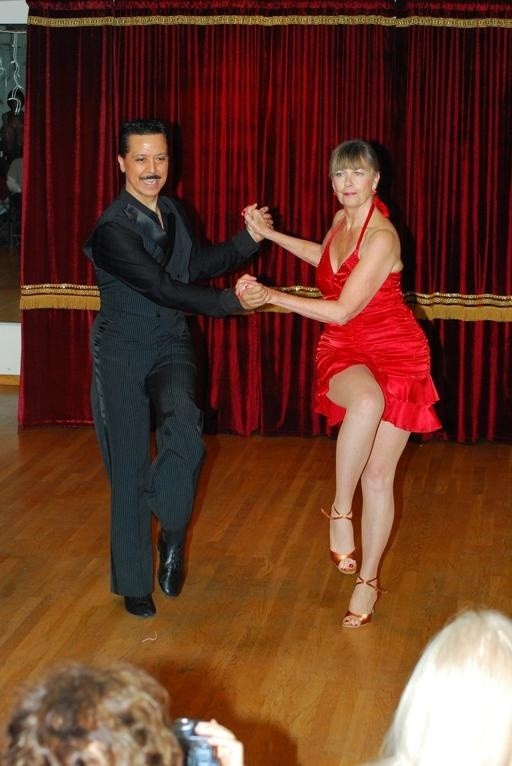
[172, 718, 220, 766]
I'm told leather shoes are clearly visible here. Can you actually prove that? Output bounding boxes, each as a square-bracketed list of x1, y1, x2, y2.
[124, 594, 157, 618]
[157, 528, 184, 598]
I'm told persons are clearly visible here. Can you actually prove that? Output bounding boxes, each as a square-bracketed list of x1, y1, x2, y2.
[80, 114, 276, 617]
[230, 137, 445, 629]
[4, 660, 248, 766]
[371, 604, 512, 766]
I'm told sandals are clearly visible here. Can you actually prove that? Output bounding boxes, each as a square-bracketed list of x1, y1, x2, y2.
[342, 576, 382, 630]
[320, 501, 358, 575]
